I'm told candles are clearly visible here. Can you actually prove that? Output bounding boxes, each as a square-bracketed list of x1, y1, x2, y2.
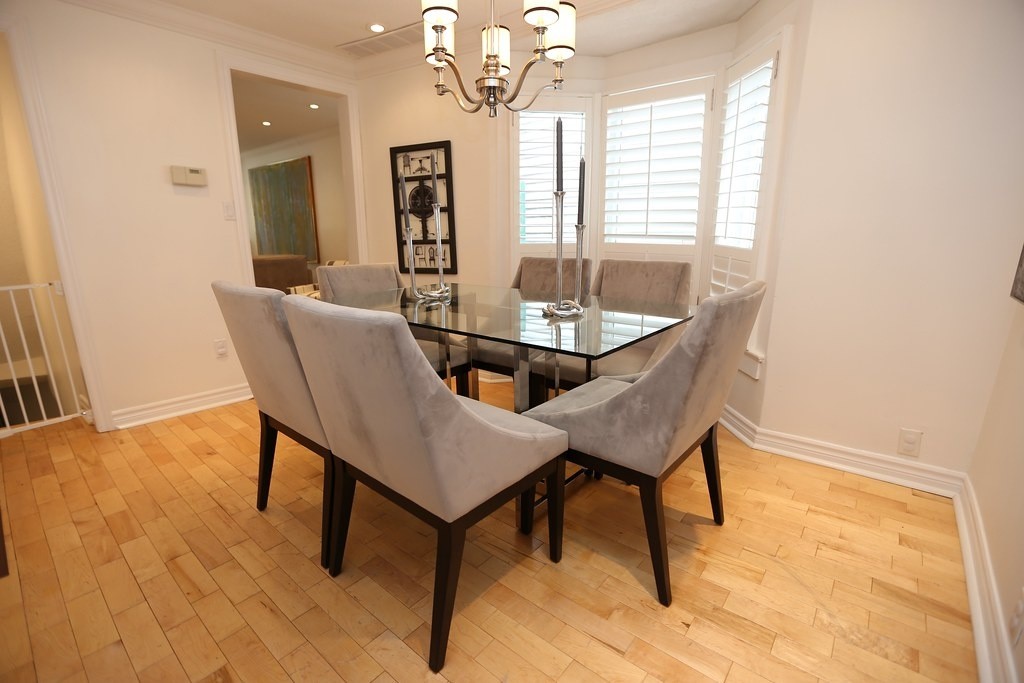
[557, 116, 563, 191]
[578, 156, 585, 224]
[400, 170, 410, 228]
[430, 150, 438, 204]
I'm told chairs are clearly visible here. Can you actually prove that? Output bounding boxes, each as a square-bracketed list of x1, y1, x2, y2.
[210, 279, 334, 569]
[279, 294, 568, 673]
[402, 154, 410, 174]
[418, 246, 446, 267]
[519, 279, 767, 608]
[459, 256, 593, 413]
[531, 259, 691, 481]
[317, 262, 473, 399]
[252, 254, 311, 294]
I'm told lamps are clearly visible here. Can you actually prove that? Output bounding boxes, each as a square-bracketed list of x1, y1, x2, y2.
[421, 0, 577, 117]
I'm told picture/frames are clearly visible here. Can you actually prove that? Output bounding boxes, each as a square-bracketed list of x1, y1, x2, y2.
[247, 155, 321, 264]
[389, 140, 458, 274]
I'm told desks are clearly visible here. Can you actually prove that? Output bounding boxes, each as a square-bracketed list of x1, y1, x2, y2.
[314, 282, 700, 563]
[410, 155, 429, 172]
